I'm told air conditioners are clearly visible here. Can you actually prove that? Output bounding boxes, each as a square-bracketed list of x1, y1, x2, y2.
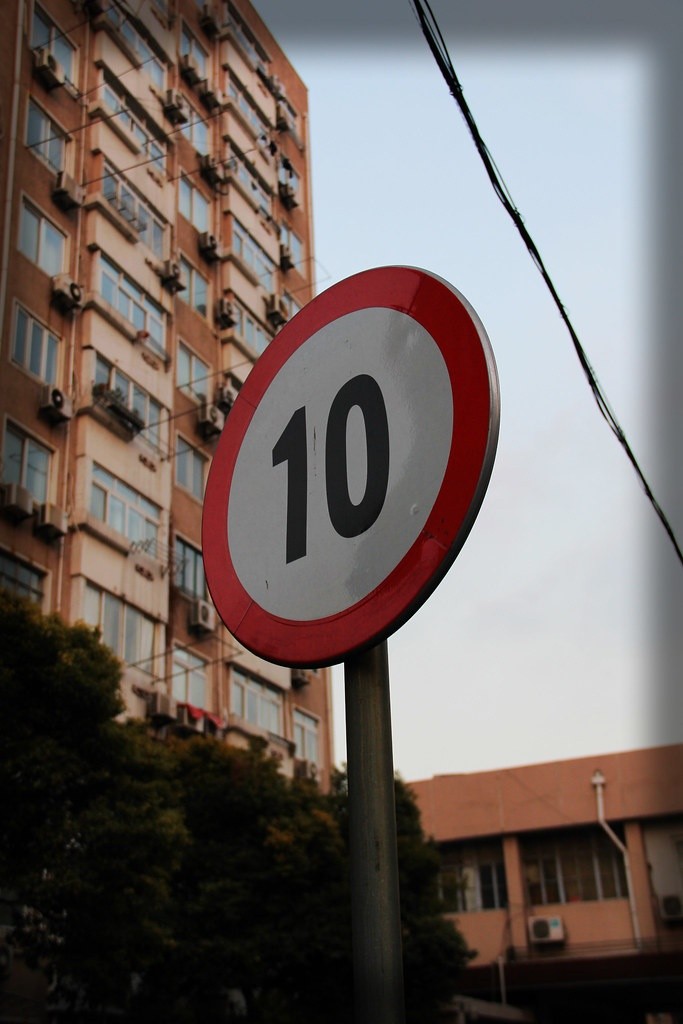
[36, 47, 67, 91]
[55, 170, 84, 207]
[219, 297, 239, 326]
[300, 759, 322, 782]
[269, 293, 288, 325]
[40, 382, 73, 422]
[527, 914, 565, 942]
[202, 154, 225, 183]
[220, 382, 240, 410]
[167, 88, 190, 124]
[178, 705, 205, 733]
[185, 53, 201, 81]
[190, 599, 216, 634]
[199, 230, 222, 263]
[292, 669, 312, 686]
[277, 101, 296, 130]
[198, 402, 224, 433]
[53, 275, 84, 310]
[658, 893, 683, 920]
[148, 691, 178, 719]
[203, 13, 222, 35]
[163, 258, 187, 291]
[38, 503, 68, 536]
[285, 183, 299, 208]
[281, 245, 296, 269]
[5, 483, 34, 515]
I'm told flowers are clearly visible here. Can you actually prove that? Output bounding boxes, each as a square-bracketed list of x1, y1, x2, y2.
[92, 382, 109, 399]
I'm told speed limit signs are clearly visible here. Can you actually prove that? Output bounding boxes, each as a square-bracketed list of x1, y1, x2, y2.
[203, 264, 491, 667]
